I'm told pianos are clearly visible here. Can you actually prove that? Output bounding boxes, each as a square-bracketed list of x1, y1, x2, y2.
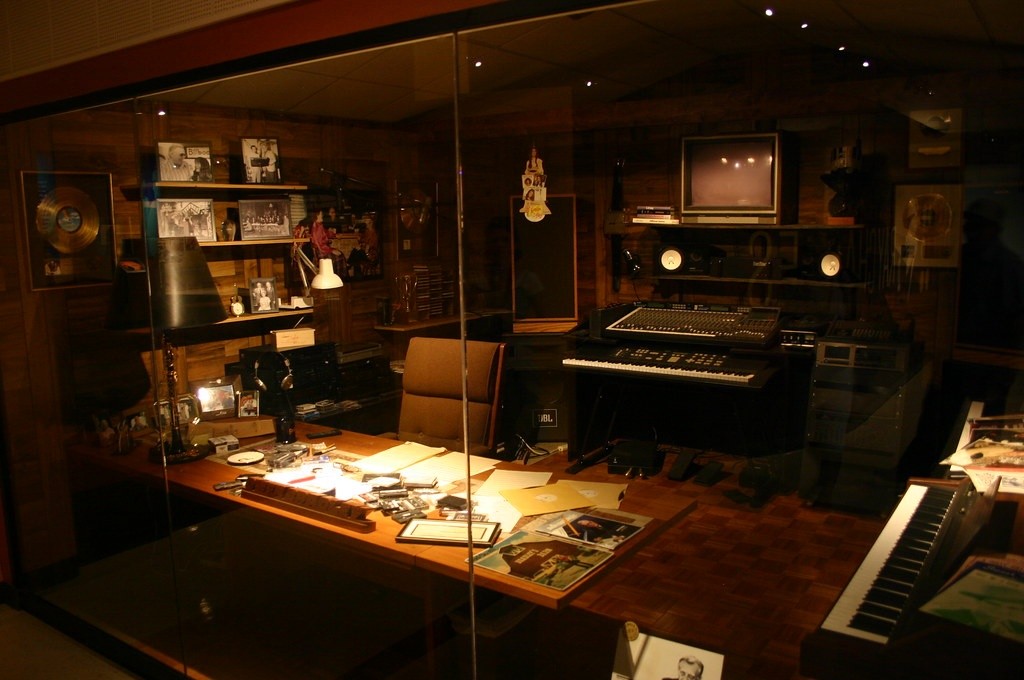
[798, 476, 1004, 680]
[552, 340, 783, 507]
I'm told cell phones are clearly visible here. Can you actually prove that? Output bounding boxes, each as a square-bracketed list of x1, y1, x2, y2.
[307, 430, 343, 439]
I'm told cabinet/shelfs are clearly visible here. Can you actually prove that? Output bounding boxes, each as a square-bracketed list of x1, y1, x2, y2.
[120, 176, 313, 338]
[642, 220, 875, 292]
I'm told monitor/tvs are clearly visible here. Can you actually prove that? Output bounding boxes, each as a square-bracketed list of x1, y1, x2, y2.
[680, 132, 783, 224]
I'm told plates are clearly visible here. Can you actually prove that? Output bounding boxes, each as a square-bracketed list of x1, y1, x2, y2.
[228, 451, 264, 464]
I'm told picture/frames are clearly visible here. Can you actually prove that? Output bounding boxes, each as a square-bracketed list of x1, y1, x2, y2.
[239, 136, 282, 184]
[246, 276, 279, 314]
[151, 394, 197, 428]
[238, 199, 294, 242]
[155, 139, 216, 183]
[153, 196, 218, 243]
[239, 390, 259, 417]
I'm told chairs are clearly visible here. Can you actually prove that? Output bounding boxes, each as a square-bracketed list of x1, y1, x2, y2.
[373, 336, 508, 460]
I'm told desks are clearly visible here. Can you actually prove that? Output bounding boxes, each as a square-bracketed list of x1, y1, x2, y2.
[57, 415, 700, 679]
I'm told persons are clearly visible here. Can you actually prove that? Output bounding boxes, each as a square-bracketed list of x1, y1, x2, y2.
[578, 518, 640, 542]
[662, 654, 705, 680]
[551, 555, 594, 570]
[311, 206, 341, 267]
[221, 391, 234, 408]
[248, 145, 263, 183]
[261, 143, 277, 183]
[241, 209, 289, 236]
[254, 281, 274, 311]
[159, 144, 194, 181]
[191, 156, 213, 181]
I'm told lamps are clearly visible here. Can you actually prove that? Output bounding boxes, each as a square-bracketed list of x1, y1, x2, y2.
[288, 245, 344, 311]
[104, 235, 228, 466]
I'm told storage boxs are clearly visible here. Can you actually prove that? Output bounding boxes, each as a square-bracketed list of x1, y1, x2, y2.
[207, 435, 237, 454]
